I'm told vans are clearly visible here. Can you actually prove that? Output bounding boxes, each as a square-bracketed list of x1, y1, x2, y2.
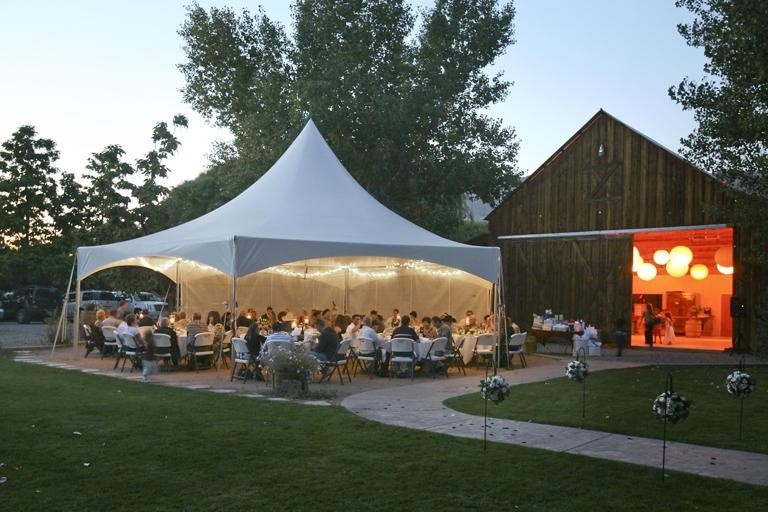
[1, 285, 63, 324]
[61, 290, 119, 322]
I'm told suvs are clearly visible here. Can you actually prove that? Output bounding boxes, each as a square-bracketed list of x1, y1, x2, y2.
[121, 290, 169, 318]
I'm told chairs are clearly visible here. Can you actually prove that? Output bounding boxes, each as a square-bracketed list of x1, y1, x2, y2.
[84, 321, 234, 374]
[231, 320, 351, 385]
[340, 324, 529, 379]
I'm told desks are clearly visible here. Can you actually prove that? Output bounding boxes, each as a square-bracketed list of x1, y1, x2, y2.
[527, 325, 574, 356]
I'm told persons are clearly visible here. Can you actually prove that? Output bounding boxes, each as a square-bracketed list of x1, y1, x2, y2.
[664, 311, 674, 345]
[652, 309, 663, 343]
[639, 304, 653, 347]
[94, 300, 520, 380]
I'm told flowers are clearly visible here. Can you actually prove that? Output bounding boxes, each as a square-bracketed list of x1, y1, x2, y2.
[722, 370, 754, 397]
[563, 359, 591, 384]
[477, 373, 514, 408]
[651, 388, 691, 425]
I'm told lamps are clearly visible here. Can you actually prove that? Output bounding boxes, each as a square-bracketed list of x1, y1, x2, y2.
[632, 242, 734, 283]
